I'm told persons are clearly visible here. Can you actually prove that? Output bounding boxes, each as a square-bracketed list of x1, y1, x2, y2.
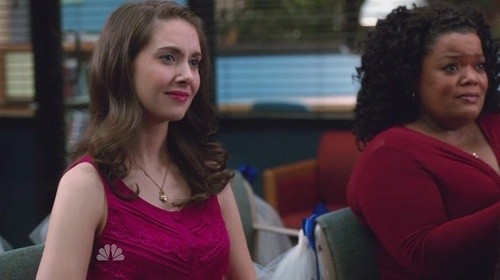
[36, 0, 257, 280]
[346, 2, 500, 280]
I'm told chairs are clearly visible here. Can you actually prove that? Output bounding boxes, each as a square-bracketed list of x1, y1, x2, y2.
[316, 208, 381, 279]
[265, 159, 327, 232]
[0, 167, 255, 280]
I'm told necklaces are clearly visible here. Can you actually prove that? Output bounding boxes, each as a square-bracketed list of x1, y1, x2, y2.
[126, 151, 173, 203]
